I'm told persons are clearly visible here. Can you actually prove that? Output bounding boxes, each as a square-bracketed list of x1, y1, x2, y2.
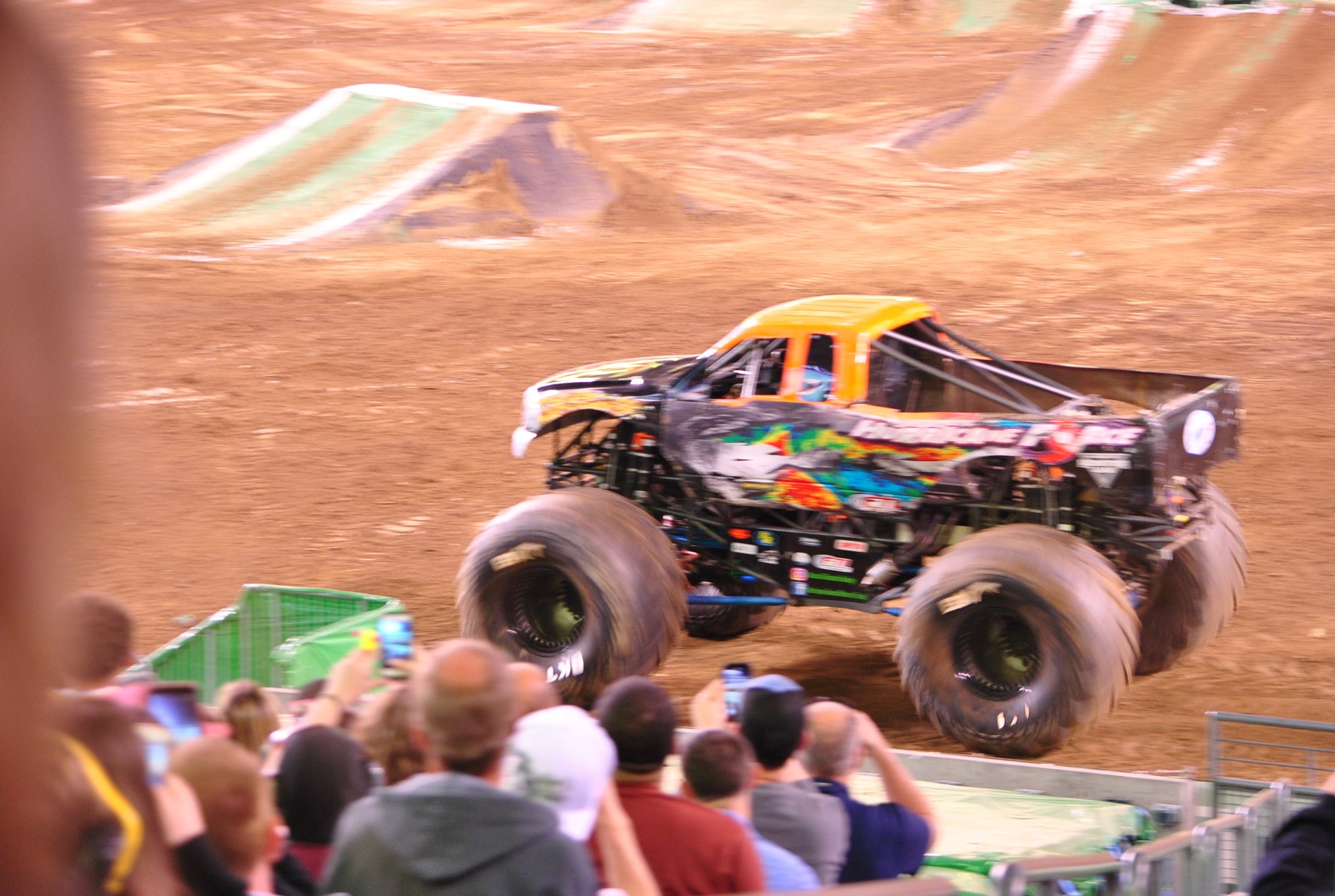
[36, 586, 960, 896]
[1230, 775, 1334, 896]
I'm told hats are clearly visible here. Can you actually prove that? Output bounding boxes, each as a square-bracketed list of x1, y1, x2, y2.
[502, 705, 618, 844]
[275, 725, 375, 846]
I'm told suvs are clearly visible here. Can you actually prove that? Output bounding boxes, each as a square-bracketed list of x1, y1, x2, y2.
[460, 291, 1254, 757]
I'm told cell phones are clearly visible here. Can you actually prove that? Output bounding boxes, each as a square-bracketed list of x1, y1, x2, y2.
[123, 720, 174, 788]
[722, 664, 751, 723]
[378, 613, 414, 678]
[146, 684, 200, 745]
